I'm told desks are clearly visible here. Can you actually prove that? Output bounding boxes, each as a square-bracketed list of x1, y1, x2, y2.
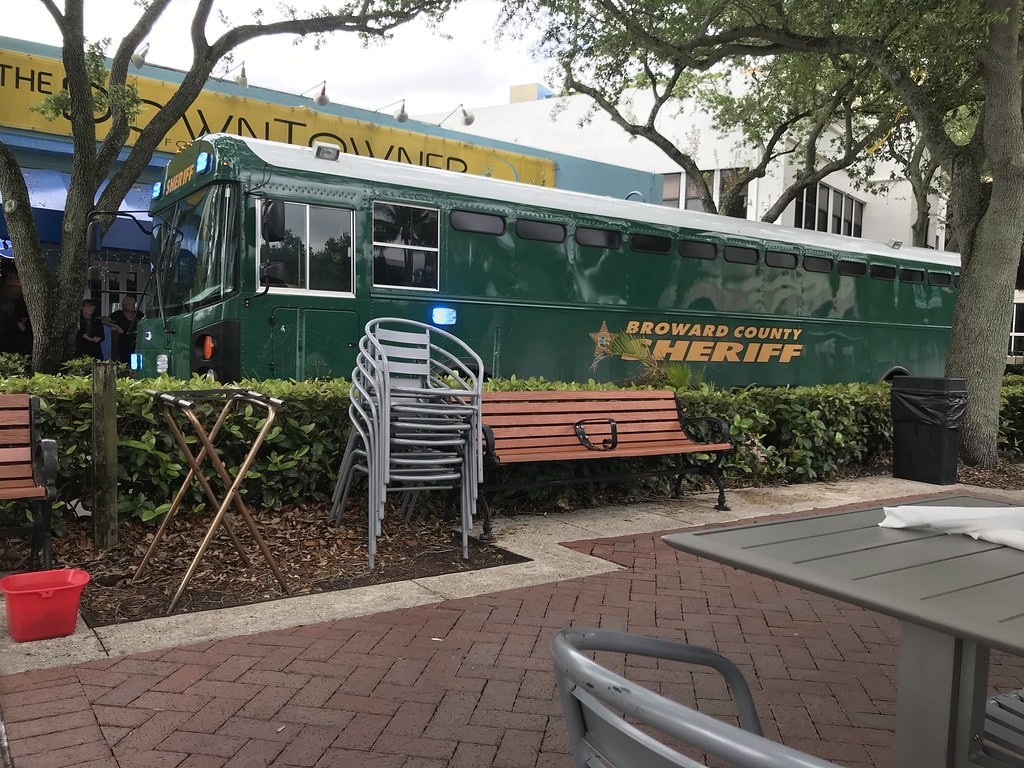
[128, 389, 286, 616]
[660, 495, 1024, 768]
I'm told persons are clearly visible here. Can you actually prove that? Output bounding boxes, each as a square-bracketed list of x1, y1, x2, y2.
[103, 297, 144, 360]
[0, 304, 33, 355]
[77, 300, 106, 361]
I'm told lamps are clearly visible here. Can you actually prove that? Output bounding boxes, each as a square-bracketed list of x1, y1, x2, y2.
[130, 43, 150, 69]
[439, 104, 474, 126]
[377, 99, 409, 123]
[219, 61, 249, 88]
[301, 81, 329, 106]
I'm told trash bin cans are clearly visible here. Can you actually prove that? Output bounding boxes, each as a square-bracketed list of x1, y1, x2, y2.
[890, 376, 968, 486]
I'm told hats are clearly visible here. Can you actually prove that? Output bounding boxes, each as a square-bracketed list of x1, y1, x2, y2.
[81, 299, 96, 305]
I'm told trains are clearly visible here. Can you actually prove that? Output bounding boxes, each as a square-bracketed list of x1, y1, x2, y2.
[126, 132, 960, 380]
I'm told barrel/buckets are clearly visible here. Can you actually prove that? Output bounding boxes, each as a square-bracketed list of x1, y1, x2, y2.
[0, 569, 90, 642]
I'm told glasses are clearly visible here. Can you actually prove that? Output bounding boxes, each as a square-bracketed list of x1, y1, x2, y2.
[85, 306, 95, 309]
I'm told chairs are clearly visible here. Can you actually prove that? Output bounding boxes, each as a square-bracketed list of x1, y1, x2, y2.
[550, 627, 1024, 768]
[332, 317, 484, 570]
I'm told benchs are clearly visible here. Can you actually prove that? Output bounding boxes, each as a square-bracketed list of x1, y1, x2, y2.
[0, 393, 60, 572]
[444, 390, 737, 546]
